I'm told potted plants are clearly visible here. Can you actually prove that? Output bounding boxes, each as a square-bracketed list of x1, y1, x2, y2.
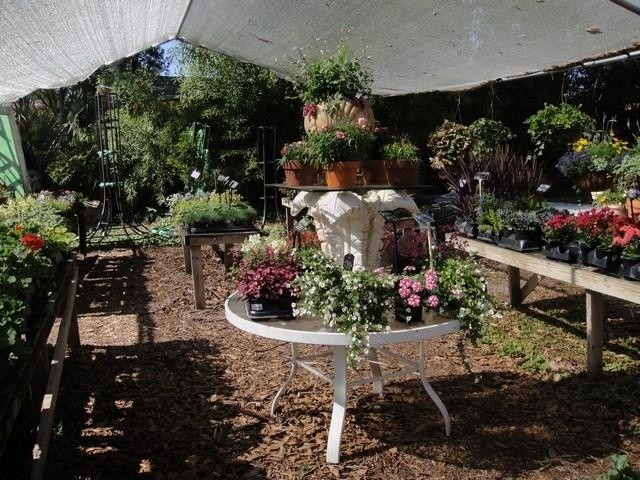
[175, 183, 228, 232]
[284, 26, 378, 132]
[227, 233, 303, 321]
[358, 133, 421, 185]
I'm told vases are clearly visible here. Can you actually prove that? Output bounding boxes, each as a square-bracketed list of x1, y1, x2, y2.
[395, 296, 423, 325]
[321, 161, 363, 188]
[282, 160, 317, 186]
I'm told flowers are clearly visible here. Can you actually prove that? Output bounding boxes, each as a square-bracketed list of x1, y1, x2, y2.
[281, 141, 303, 156]
[398, 266, 440, 324]
[12, 220, 46, 258]
[449, 265, 495, 331]
[303, 104, 318, 118]
[336, 130, 346, 140]
[290, 249, 397, 362]
[540, 200, 640, 262]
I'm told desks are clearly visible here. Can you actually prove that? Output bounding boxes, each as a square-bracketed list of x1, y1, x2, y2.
[178, 227, 266, 312]
[441, 226, 640, 386]
